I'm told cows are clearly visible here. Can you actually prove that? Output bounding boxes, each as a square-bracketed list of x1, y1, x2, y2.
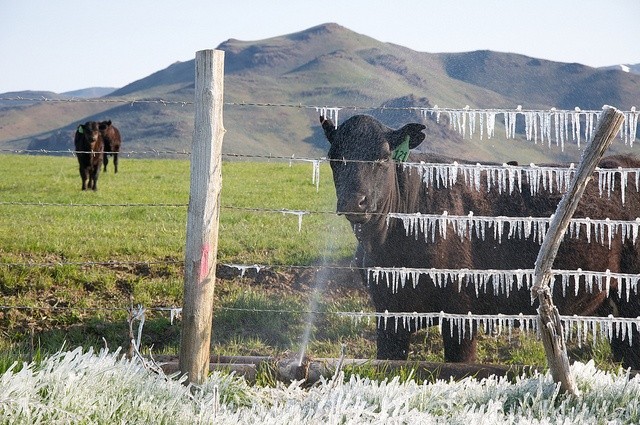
[74, 122, 105, 192]
[320, 113, 640, 371]
[100, 120, 121, 174]
